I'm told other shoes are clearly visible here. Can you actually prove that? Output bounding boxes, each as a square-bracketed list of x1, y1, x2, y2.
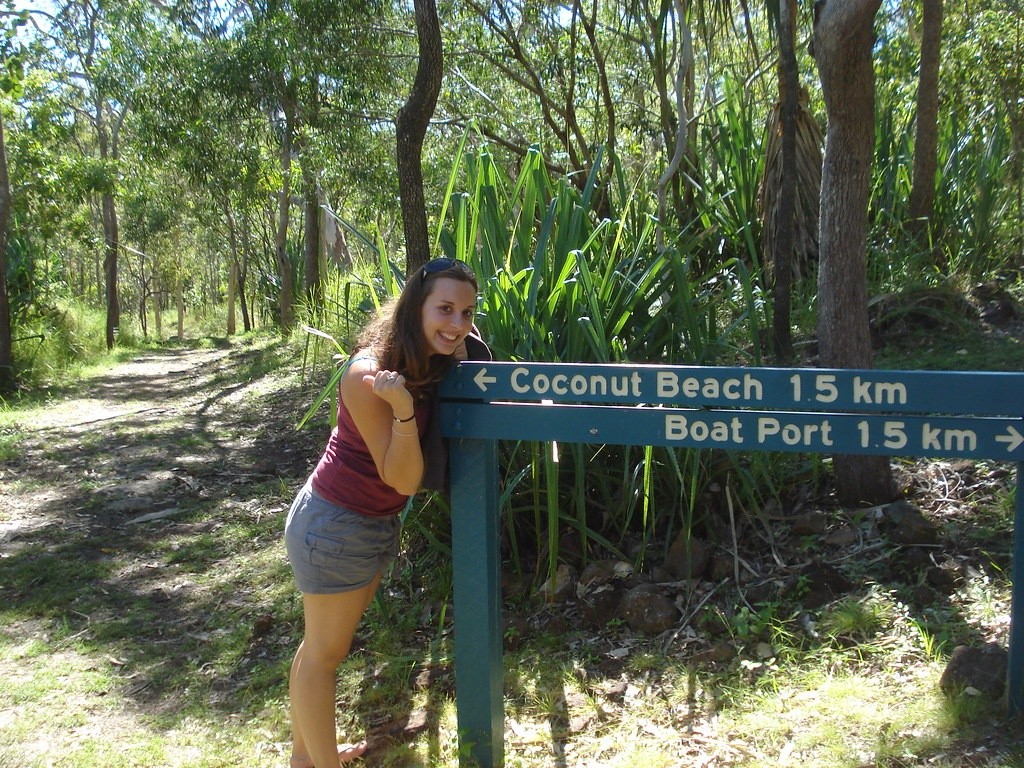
[290, 740, 368, 768]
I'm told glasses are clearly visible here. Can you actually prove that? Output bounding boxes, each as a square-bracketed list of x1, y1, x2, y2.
[422, 258, 469, 279]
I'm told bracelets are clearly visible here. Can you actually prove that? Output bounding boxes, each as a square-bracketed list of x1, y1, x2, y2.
[393, 413, 415, 422]
[391, 427, 419, 437]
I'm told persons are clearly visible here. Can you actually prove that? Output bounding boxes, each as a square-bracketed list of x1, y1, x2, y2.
[284, 258, 477, 768]
